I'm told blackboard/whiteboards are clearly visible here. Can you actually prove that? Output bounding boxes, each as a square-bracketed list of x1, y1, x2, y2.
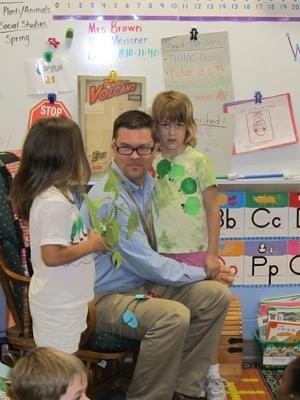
[0, 0, 300, 186]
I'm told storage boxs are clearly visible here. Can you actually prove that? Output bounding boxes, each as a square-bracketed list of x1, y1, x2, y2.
[217, 296, 243, 375]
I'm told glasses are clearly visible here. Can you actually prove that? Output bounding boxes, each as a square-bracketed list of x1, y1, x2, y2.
[113, 142, 155, 155]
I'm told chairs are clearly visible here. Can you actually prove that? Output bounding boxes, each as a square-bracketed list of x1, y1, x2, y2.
[0, 151, 140, 400]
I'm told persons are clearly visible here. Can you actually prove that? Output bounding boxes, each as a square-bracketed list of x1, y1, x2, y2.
[148, 90, 227, 399]
[277, 357, 300, 400]
[80, 110, 235, 399]
[8, 117, 112, 355]
[6, 347, 90, 400]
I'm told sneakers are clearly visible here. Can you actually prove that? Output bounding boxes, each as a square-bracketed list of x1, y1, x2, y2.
[203, 376, 228, 400]
